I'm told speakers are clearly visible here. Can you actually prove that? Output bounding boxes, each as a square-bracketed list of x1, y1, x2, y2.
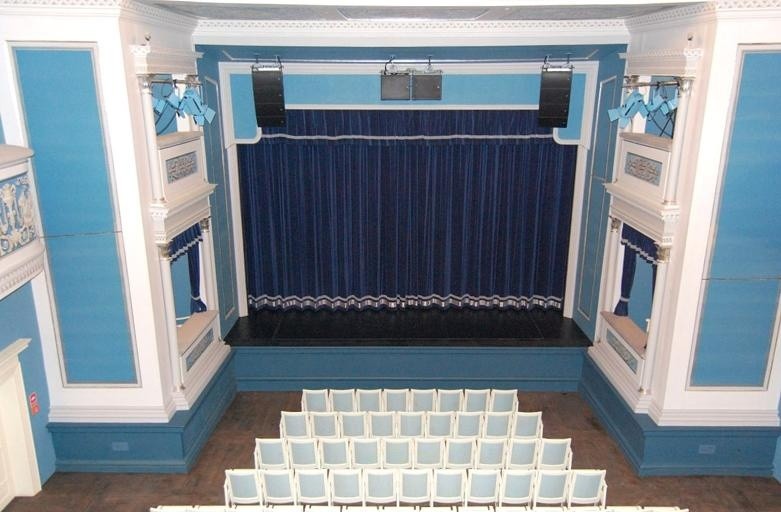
[538, 63, 573, 128]
[251, 63, 286, 128]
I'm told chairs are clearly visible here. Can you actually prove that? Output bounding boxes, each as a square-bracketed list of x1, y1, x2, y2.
[300, 388, 521, 413]
[221, 466, 609, 507]
[278, 410, 545, 438]
[149, 503, 691, 512]
[251, 436, 574, 471]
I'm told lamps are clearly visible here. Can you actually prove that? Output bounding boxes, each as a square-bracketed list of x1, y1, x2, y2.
[606, 82, 682, 130]
[154, 86, 216, 126]
